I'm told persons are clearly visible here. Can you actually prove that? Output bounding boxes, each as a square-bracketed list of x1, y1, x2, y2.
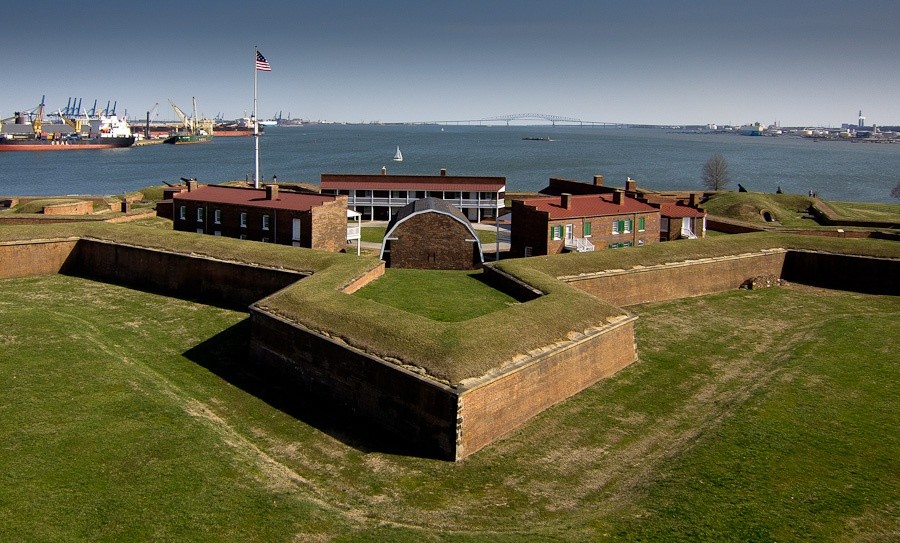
[809, 190, 816, 198]
[776, 185, 783, 194]
[738, 184, 747, 192]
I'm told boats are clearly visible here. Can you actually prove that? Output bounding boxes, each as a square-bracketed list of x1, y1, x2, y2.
[173, 133, 213, 145]
[522, 136, 550, 141]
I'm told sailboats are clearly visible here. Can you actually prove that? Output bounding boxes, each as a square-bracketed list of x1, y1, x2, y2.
[393, 145, 403, 162]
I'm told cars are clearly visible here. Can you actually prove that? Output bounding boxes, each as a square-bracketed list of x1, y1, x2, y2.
[767, 126, 830, 136]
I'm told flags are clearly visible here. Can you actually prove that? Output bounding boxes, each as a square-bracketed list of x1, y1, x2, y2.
[257, 50, 271, 71]
